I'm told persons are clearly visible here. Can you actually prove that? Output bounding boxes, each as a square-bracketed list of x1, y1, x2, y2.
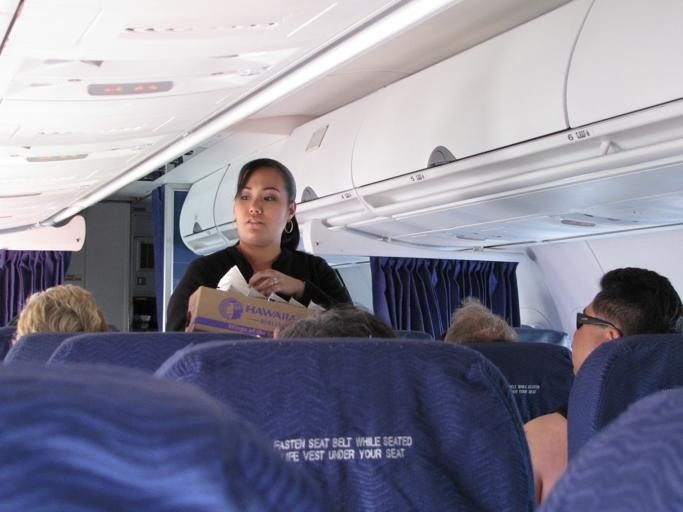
[437, 293, 518, 344]
[13, 285, 109, 351]
[163, 160, 354, 341]
[513, 266, 681, 506]
[272, 301, 401, 341]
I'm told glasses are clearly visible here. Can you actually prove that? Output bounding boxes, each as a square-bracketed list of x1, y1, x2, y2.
[577, 312, 624, 339]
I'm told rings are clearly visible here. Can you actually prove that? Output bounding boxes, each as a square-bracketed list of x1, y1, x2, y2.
[272, 276, 280, 285]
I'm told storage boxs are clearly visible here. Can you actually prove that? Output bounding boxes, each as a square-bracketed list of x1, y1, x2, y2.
[186, 284, 321, 344]
